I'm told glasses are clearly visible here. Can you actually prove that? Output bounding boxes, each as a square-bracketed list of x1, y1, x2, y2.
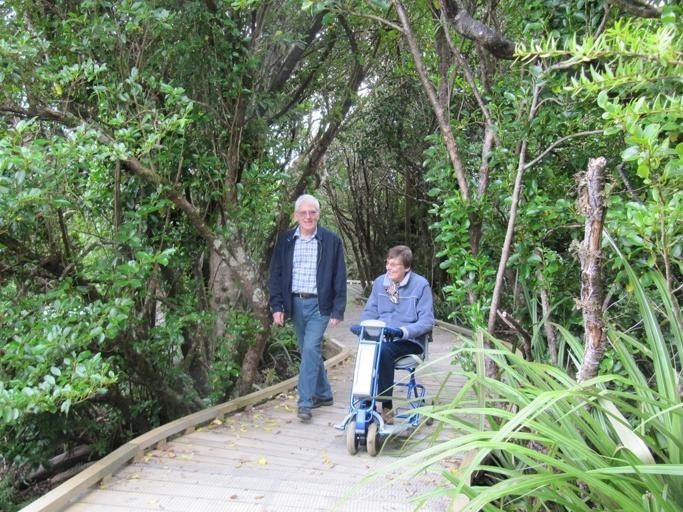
[384, 260, 404, 267]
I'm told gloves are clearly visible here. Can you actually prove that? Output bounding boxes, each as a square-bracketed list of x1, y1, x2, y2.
[351, 324, 362, 335]
[384, 327, 402, 342]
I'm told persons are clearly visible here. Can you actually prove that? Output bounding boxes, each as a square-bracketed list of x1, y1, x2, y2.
[267, 193, 348, 420]
[360, 244, 435, 425]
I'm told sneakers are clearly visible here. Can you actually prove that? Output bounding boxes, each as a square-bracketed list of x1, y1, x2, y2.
[381, 408, 394, 424]
[311, 397, 333, 407]
[297, 406, 311, 420]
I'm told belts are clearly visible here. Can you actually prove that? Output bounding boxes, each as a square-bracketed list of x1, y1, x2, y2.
[293, 292, 319, 299]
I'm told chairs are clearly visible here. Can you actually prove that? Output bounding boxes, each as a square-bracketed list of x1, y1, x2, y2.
[393, 330, 433, 385]
